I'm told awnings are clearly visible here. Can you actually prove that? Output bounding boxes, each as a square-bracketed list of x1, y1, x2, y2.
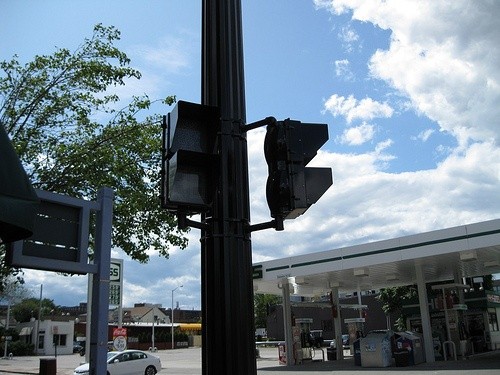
[179, 324, 201, 330]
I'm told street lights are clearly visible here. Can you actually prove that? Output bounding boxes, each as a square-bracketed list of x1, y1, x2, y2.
[171, 284, 183, 349]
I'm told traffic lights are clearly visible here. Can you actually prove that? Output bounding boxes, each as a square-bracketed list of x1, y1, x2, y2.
[265, 115, 333, 220]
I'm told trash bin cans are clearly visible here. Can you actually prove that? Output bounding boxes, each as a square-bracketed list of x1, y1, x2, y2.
[327, 348, 337, 360]
[392, 350, 412, 367]
[39, 358, 57, 375]
[471, 338, 484, 354]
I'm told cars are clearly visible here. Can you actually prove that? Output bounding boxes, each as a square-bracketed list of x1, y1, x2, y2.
[255, 328, 267, 338]
[330, 334, 350, 349]
[80, 342, 113, 356]
[73, 341, 84, 352]
[74, 350, 161, 375]
